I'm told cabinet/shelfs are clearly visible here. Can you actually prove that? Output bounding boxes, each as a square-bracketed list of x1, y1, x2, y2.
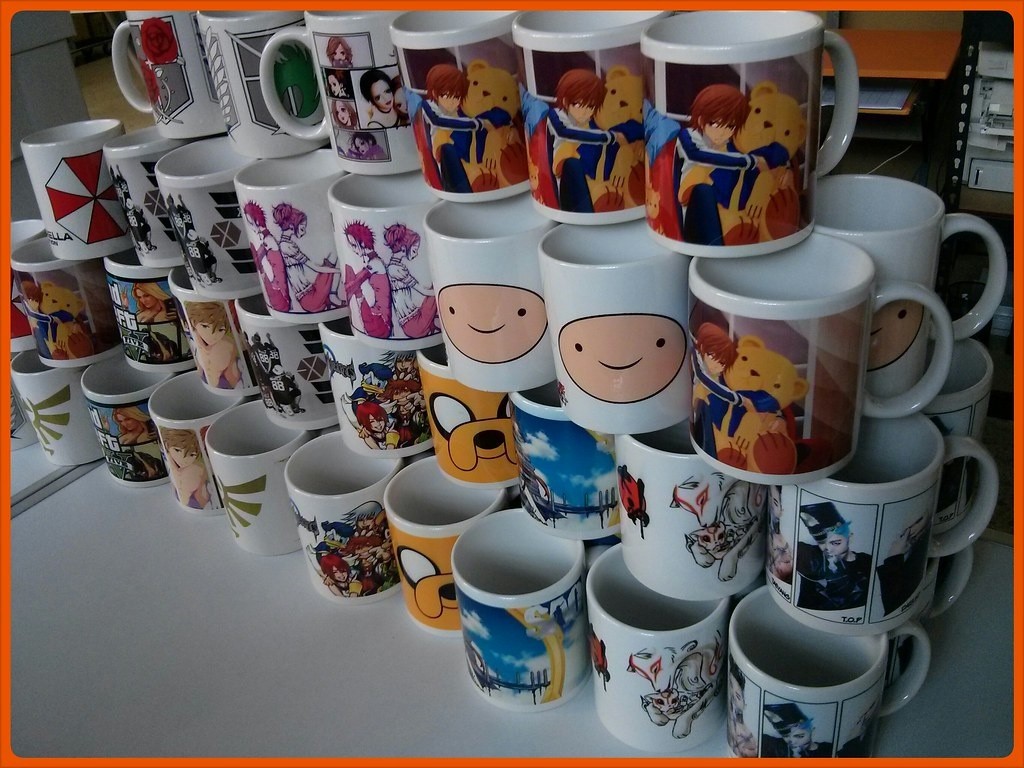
[817, 27, 983, 215]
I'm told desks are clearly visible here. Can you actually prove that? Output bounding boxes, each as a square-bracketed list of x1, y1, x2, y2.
[9, 460, 1016, 758]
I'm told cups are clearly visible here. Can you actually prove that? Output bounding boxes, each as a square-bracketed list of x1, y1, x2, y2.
[810, 175, 1007, 400]
[8, 349, 931, 757]
[918, 547, 979, 619]
[110, 11, 860, 259]
[18, 117, 802, 466]
[927, 338, 994, 537]
[10, 220, 999, 641]
